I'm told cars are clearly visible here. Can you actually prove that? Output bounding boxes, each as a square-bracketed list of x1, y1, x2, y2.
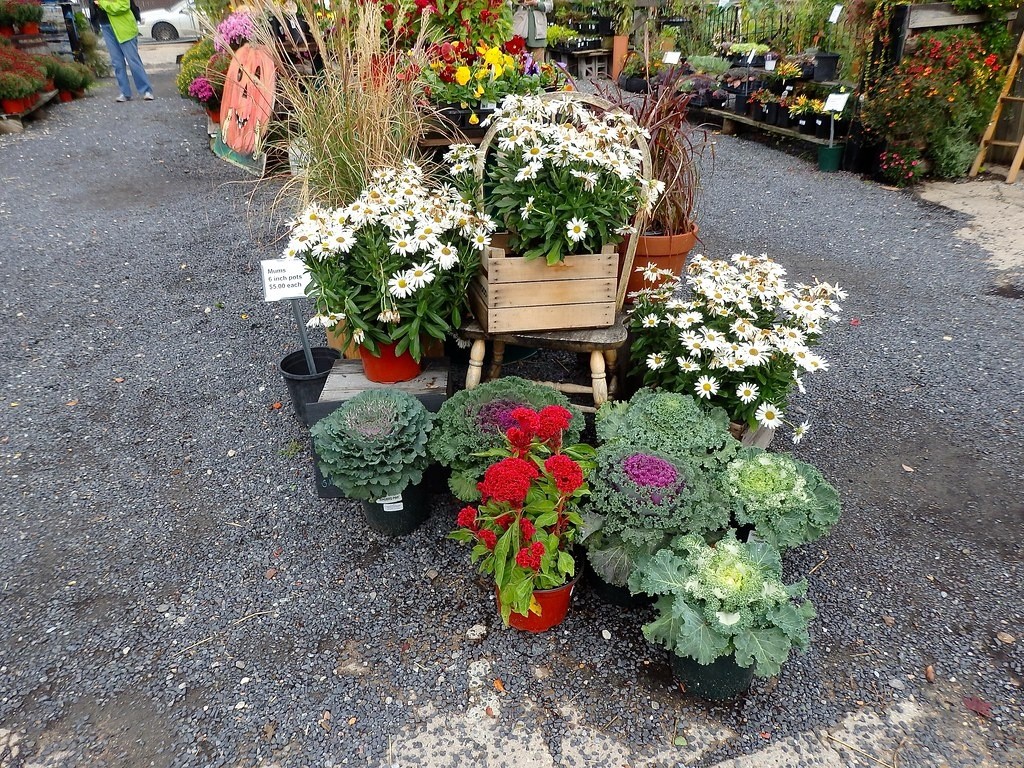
[138, 0, 209, 41]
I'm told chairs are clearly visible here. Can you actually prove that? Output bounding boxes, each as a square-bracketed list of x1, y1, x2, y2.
[465, 91, 653, 416]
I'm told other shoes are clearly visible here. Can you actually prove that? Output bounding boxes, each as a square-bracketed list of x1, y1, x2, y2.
[144, 92, 154, 100]
[116, 94, 130, 101]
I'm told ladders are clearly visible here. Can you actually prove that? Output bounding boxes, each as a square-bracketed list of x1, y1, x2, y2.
[969, 31, 1024, 184]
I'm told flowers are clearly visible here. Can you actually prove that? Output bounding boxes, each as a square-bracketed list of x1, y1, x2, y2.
[172, 0, 573, 372]
[750, 63, 843, 121]
[626, 253, 848, 440]
[0, 0, 47, 97]
[482, 94, 666, 261]
[849, 0, 1020, 183]
[448, 402, 599, 618]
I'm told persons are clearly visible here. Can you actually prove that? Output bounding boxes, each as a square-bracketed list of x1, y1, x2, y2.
[514, 0, 553, 69]
[92, 0, 154, 100]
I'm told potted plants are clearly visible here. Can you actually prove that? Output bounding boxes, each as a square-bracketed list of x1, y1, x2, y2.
[308, 387, 439, 535]
[593, 64, 715, 306]
[546, 0, 623, 53]
[428, 376, 840, 702]
[48, 59, 95, 103]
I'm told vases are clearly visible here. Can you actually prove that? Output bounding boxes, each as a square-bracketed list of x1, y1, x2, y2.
[469, 233, 618, 334]
[812, 53, 840, 82]
[494, 547, 585, 633]
[750, 102, 845, 139]
[205, 107, 220, 122]
[274, 317, 445, 425]
[289, 17, 308, 43]
[2, 20, 44, 113]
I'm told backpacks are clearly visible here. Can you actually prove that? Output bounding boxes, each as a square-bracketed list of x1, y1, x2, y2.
[130, 0, 140, 20]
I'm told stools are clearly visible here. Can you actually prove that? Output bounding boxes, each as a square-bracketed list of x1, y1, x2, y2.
[461, 323, 627, 443]
